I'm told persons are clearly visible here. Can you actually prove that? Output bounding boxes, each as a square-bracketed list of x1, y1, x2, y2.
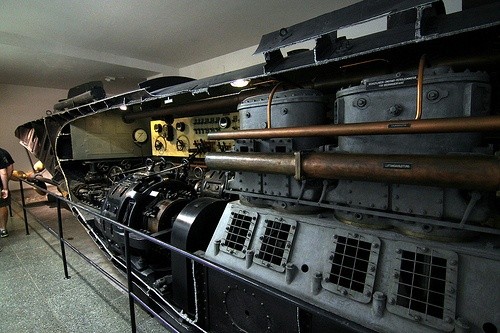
[0, 146, 16, 238]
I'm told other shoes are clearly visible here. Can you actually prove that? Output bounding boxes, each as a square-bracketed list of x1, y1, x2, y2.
[0, 228, 9, 237]
